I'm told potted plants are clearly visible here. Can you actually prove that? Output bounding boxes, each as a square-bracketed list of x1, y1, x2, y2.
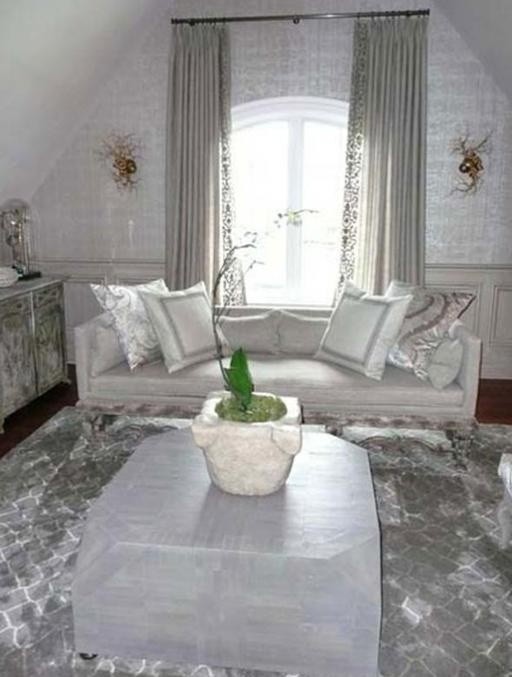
[191, 207, 318, 495]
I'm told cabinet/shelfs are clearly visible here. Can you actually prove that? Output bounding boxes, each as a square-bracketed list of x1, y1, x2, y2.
[1, 273, 72, 434]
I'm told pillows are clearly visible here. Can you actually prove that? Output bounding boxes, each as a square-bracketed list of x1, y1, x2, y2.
[311, 278, 478, 389]
[89, 277, 235, 375]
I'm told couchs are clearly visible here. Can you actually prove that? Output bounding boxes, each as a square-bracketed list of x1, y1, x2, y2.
[74, 307, 483, 474]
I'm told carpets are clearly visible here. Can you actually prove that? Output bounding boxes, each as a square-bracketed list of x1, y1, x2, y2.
[0, 405, 512, 677]
